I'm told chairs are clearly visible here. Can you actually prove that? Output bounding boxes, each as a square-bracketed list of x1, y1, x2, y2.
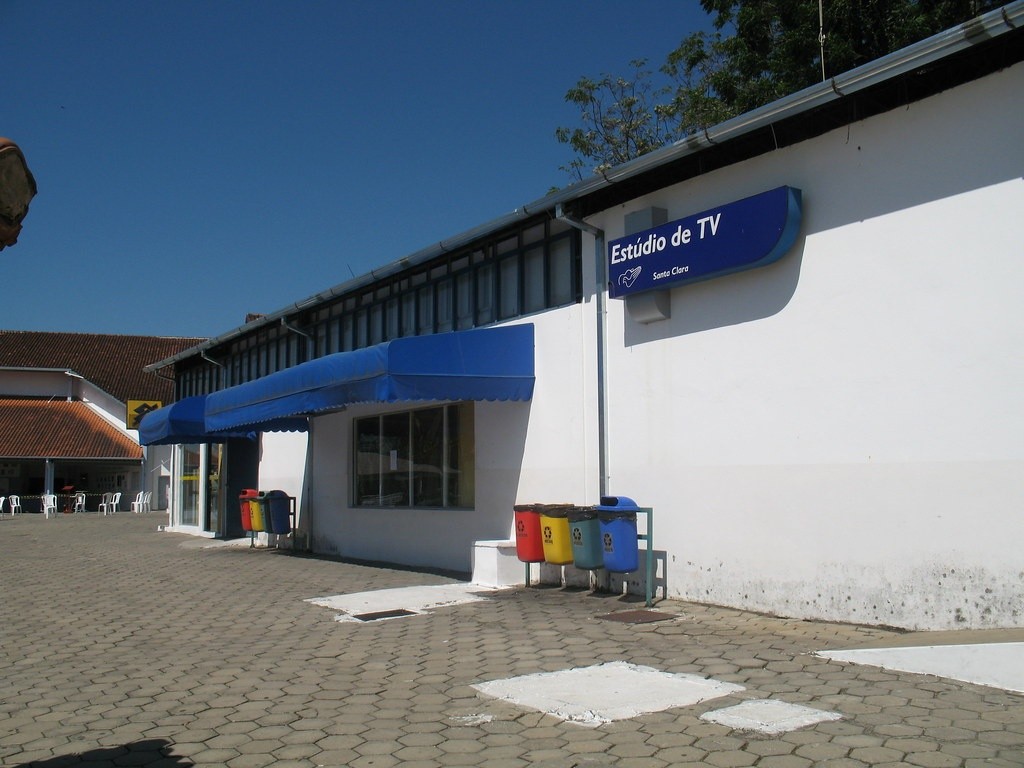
[131, 491, 153, 513]
[70, 493, 87, 514]
[0, 497, 5, 519]
[99, 492, 122, 516]
[41, 490, 58, 519]
[9, 495, 22, 516]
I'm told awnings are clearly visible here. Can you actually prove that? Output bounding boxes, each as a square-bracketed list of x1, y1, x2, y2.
[140, 322, 535, 446]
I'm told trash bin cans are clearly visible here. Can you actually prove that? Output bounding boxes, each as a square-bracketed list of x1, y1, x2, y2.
[566, 506, 605, 571]
[514, 503, 545, 563]
[239, 489, 274, 533]
[537, 504, 575, 565]
[596, 496, 640, 574]
[266, 490, 291, 535]
